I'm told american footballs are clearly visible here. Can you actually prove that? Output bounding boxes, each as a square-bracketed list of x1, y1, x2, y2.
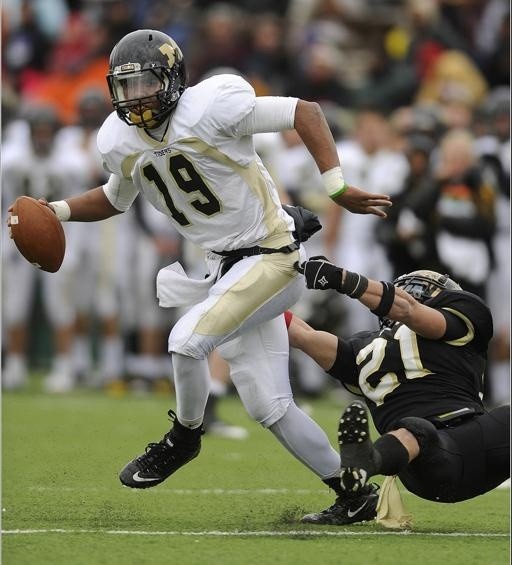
[11, 196, 65, 273]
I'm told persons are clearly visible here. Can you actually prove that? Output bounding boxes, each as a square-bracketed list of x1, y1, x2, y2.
[7, 28, 392, 526]
[285, 255, 510, 505]
[1, 0, 511, 439]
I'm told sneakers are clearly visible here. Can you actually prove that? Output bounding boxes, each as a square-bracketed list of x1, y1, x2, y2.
[299, 401, 383, 525]
[119, 428, 201, 488]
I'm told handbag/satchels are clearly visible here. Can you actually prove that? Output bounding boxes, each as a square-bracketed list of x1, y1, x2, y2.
[282, 204, 322, 242]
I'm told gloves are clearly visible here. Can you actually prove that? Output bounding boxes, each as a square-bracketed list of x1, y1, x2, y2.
[293, 256, 343, 290]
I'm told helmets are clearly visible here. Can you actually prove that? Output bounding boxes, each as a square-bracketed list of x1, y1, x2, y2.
[379, 270, 462, 328]
[106, 29, 185, 112]
